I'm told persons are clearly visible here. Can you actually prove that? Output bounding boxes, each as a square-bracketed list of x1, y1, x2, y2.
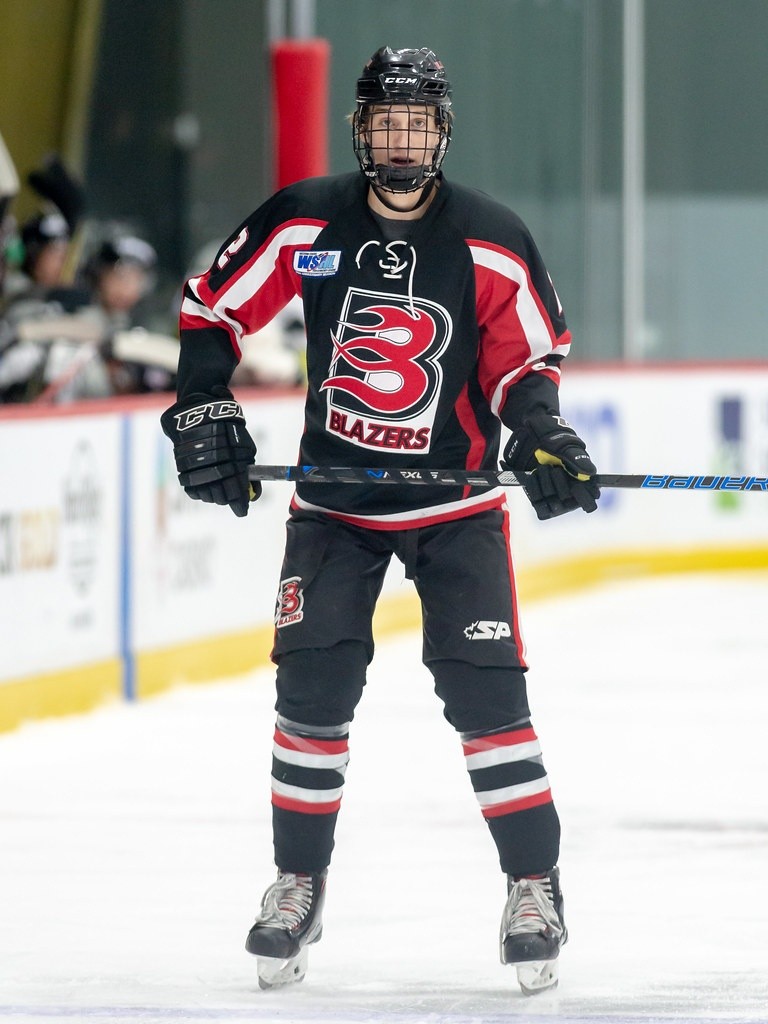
[0, 129, 309, 405]
[162, 43, 604, 996]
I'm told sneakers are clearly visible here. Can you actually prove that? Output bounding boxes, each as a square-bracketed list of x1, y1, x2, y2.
[500, 865, 568, 996]
[245, 867, 328, 991]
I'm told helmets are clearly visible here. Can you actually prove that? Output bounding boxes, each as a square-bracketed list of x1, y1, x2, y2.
[352, 45, 454, 195]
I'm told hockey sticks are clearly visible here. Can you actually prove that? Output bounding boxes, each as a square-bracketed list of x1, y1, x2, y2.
[245, 459, 768, 498]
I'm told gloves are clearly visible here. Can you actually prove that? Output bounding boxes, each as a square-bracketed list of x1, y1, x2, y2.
[499, 415, 601, 520]
[160, 385, 262, 517]
